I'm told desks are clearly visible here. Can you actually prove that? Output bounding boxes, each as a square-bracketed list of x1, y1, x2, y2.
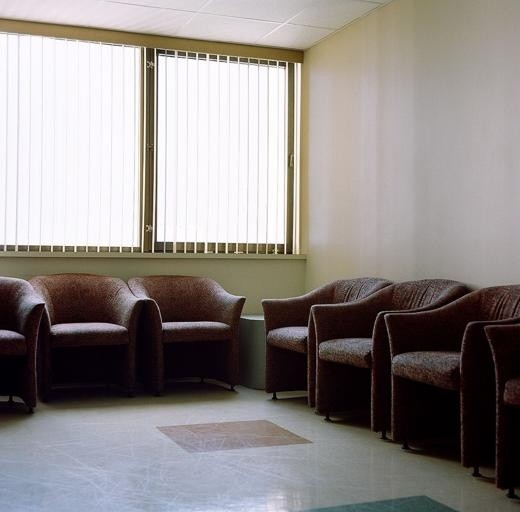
[239, 313, 270, 394]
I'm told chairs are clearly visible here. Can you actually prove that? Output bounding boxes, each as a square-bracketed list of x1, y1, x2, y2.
[311, 271, 466, 445]
[125, 269, 249, 400]
[259, 269, 388, 417]
[1, 270, 43, 416]
[26, 270, 149, 408]
[475, 314, 520, 505]
[375, 281, 520, 475]
